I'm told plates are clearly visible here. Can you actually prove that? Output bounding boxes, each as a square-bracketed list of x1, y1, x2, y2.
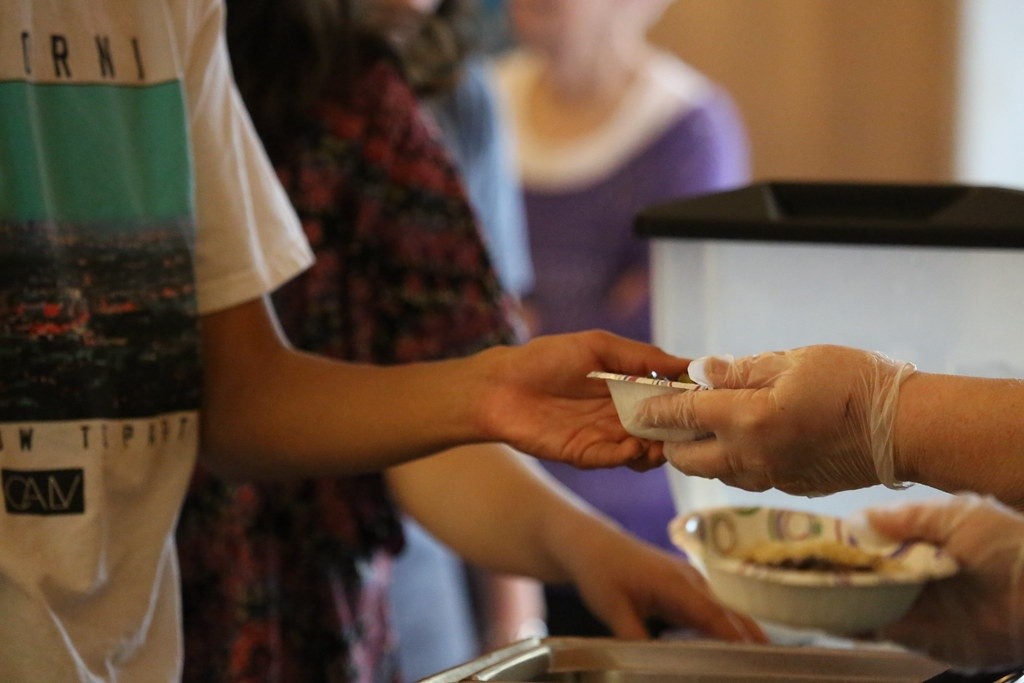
[588, 372, 717, 442]
[668, 507, 958, 630]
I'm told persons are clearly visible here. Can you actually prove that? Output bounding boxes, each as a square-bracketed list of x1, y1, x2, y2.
[0, 0, 695, 683]
[621, 340, 1024, 671]
[392, 0, 536, 683]
[175, 0, 768, 683]
[490, 0, 754, 558]
